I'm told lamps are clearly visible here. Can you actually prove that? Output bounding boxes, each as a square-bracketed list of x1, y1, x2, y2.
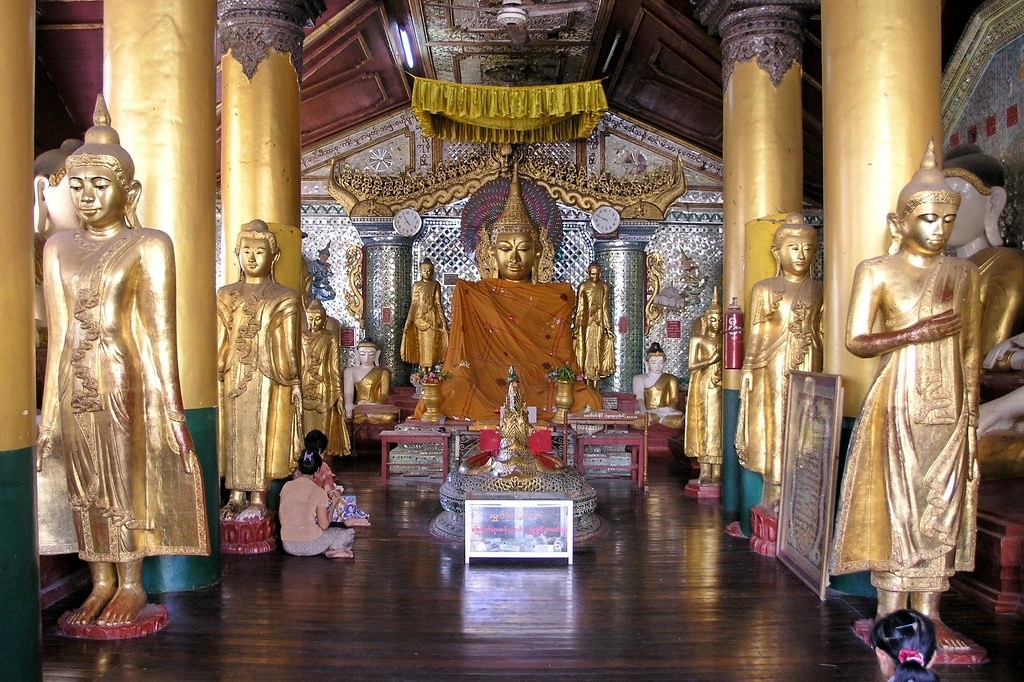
[601, 30, 622, 73]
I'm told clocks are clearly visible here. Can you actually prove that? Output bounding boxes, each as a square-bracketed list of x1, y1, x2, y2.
[392, 209, 422, 237]
[591, 205, 620, 234]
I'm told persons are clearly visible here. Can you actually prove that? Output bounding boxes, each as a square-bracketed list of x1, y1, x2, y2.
[976, 332, 1024, 481]
[216, 218, 302, 521]
[400, 258, 448, 372]
[343, 338, 397, 424]
[575, 261, 615, 380]
[733, 213, 824, 511]
[37, 97, 209, 627]
[34, 139, 81, 349]
[633, 342, 684, 428]
[299, 300, 351, 456]
[279, 450, 355, 558]
[870, 609, 940, 682]
[940, 142, 1024, 366]
[293, 429, 371, 526]
[413, 214, 603, 425]
[826, 167, 980, 651]
[683, 302, 722, 485]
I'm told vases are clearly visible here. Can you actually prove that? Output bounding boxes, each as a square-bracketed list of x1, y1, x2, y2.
[550, 380, 573, 424]
[420, 382, 443, 422]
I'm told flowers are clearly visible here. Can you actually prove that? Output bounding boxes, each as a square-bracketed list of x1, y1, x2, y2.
[409, 367, 453, 386]
[543, 361, 585, 386]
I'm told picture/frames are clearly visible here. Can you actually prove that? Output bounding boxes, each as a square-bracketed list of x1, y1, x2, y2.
[776, 369, 843, 601]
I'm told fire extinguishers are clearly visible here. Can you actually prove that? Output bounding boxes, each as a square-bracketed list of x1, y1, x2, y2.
[722, 296, 744, 369]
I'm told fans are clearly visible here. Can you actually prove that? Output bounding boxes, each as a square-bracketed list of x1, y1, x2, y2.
[421, 1, 591, 45]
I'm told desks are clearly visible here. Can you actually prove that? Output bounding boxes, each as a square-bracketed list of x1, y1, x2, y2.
[573, 433, 644, 488]
[352, 405, 400, 448]
[378, 430, 451, 485]
[406, 419, 473, 473]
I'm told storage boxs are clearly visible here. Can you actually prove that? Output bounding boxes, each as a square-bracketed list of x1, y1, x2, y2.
[463, 492, 573, 565]
